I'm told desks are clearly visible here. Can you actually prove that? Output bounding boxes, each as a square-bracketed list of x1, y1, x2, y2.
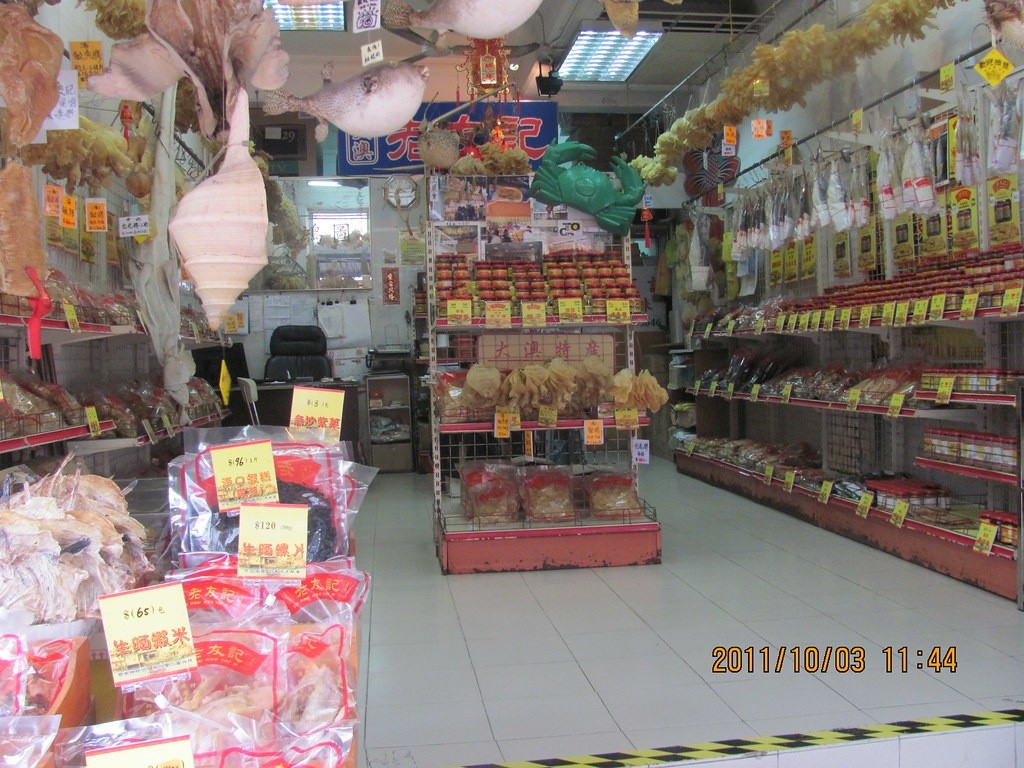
[193, 379, 362, 463]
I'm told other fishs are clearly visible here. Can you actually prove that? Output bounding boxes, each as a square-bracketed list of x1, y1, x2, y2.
[381, 0, 544, 40]
[261, 61, 430, 145]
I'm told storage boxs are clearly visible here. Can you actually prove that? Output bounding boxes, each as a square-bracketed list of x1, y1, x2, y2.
[641, 353, 669, 372]
[670, 409, 696, 429]
[654, 254, 670, 297]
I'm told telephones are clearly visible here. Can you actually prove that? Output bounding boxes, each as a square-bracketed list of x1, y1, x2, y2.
[368, 342, 411, 354]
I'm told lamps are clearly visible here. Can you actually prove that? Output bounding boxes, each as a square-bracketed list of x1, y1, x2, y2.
[555, 20, 664, 85]
[536, 76, 564, 98]
[261, 0, 348, 32]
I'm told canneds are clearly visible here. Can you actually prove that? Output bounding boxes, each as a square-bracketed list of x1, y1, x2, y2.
[920, 367, 1024, 394]
[979, 511, 1018, 547]
[865, 478, 951, 515]
[922, 429, 1017, 473]
[436, 253, 642, 315]
[769, 241, 1024, 326]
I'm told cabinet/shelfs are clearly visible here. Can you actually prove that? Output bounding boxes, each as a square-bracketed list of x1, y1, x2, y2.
[365, 373, 415, 474]
[664, 39, 1024, 612]
[410, 173, 663, 574]
[0, 29, 234, 477]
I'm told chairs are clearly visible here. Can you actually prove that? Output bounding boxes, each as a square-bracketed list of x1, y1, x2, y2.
[263, 325, 331, 380]
